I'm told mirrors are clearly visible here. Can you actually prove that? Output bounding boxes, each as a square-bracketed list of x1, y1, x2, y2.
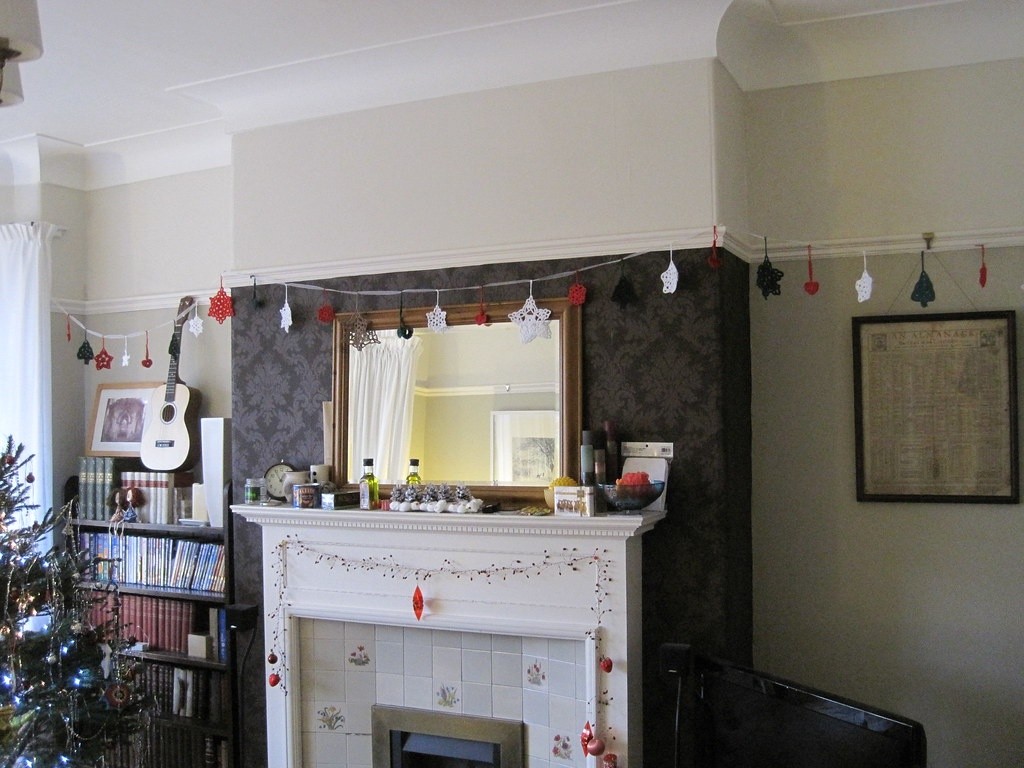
[332, 298, 583, 502]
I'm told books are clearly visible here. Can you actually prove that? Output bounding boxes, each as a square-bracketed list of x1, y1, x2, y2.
[101, 722, 228, 768]
[122, 471, 210, 525]
[77, 455, 136, 521]
[79, 532, 225, 592]
[113, 656, 221, 723]
[81, 588, 219, 659]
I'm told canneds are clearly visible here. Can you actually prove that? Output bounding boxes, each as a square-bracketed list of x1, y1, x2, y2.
[292, 483, 320, 508]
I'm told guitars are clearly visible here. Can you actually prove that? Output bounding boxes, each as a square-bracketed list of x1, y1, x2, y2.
[138, 295, 204, 472]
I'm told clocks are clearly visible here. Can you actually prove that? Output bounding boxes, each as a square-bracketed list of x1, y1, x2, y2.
[264, 462, 299, 502]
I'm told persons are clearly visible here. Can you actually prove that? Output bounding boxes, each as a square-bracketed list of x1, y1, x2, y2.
[108, 487, 142, 538]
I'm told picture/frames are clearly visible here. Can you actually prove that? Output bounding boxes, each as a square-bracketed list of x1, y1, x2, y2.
[491, 410, 559, 488]
[851, 310, 1019, 505]
[81, 380, 168, 458]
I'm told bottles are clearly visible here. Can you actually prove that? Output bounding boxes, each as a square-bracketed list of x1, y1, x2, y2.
[244, 477, 267, 505]
[283, 471, 310, 503]
[359, 458, 379, 510]
[406, 459, 421, 485]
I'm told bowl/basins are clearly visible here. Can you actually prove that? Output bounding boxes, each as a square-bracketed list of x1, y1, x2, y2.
[597, 479, 665, 510]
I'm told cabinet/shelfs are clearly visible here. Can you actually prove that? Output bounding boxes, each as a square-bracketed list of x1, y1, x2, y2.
[63, 475, 232, 768]
